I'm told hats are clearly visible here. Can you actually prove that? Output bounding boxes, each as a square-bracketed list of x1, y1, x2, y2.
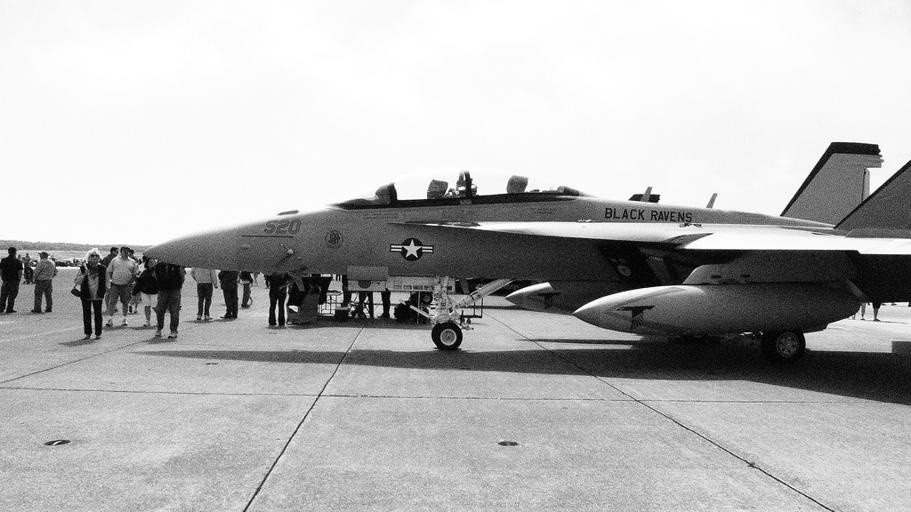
[38, 252, 48, 257]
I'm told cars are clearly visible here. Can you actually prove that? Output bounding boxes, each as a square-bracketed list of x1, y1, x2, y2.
[33, 258, 90, 268]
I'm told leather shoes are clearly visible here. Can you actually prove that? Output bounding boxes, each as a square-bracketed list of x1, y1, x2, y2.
[6, 310, 17, 313]
[45, 310, 52, 312]
[31, 309, 41, 313]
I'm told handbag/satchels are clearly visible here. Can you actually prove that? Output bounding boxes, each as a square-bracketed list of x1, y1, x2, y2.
[219, 273, 235, 291]
[239, 273, 250, 281]
[70, 283, 80, 297]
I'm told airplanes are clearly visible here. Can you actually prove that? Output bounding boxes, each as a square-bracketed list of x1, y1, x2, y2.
[136, 141, 911, 366]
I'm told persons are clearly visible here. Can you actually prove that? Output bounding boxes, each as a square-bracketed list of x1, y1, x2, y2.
[240, 271, 254, 308]
[24, 263, 34, 285]
[378, 291, 391, 320]
[264, 273, 289, 327]
[103, 246, 159, 329]
[24, 252, 30, 267]
[873, 302, 882, 322]
[0, 246, 24, 314]
[18, 255, 22, 261]
[72, 247, 107, 340]
[218, 270, 240, 320]
[31, 251, 59, 313]
[190, 267, 219, 321]
[154, 262, 186, 339]
[350, 291, 368, 321]
[861, 303, 867, 319]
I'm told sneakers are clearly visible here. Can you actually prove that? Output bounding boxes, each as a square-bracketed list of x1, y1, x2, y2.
[106, 319, 113, 327]
[144, 320, 151, 327]
[168, 333, 179, 338]
[121, 319, 128, 326]
[155, 330, 162, 336]
[197, 314, 202, 321]
[204, 315, 214, 320]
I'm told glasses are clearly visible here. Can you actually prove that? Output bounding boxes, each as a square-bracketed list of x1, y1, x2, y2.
[89, 254, 99, 257]
